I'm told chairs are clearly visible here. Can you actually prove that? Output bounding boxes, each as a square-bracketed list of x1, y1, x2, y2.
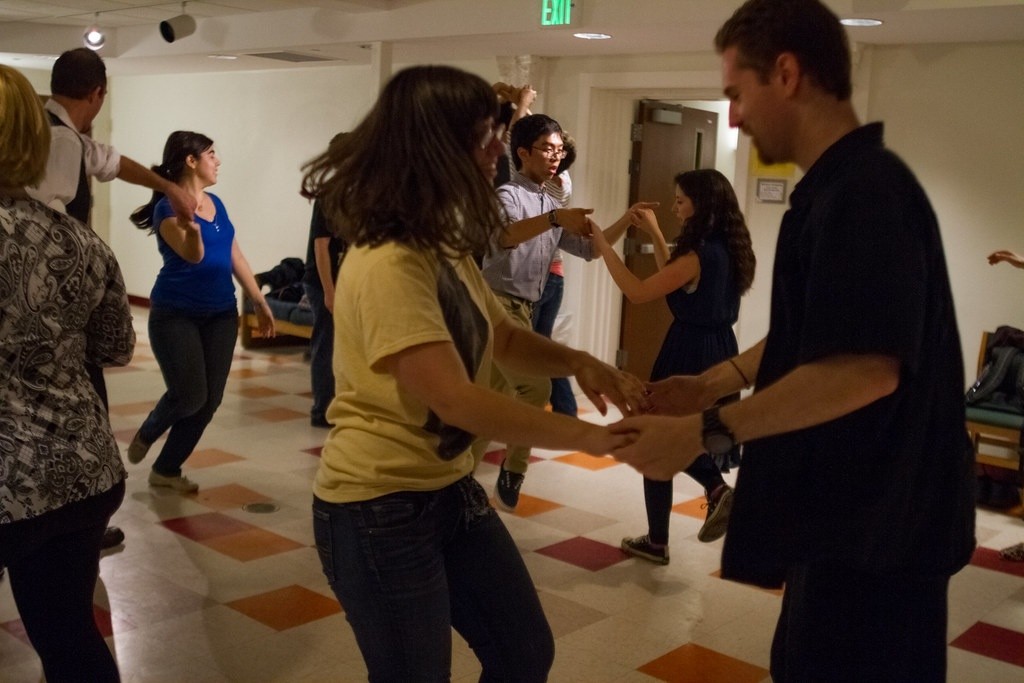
[964, 328, 1024, 498]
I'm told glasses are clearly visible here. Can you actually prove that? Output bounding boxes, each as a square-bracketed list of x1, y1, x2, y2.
[465, 125, 505, 149]
[526, 145, 568, 159]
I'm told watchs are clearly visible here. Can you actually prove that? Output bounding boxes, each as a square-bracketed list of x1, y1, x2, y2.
[701, 405, 737, 456]
[547, 209, 560, 229]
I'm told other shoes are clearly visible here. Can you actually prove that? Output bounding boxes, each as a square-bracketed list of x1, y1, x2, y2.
[311, 417, 333, 426]
[148, 471, 197, 493]
[129, 430, 150, 466]
[103, 526, 125, 550]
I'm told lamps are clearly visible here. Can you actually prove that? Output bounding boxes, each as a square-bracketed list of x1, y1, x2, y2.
[160, 2, 197, 43]
[82, 25, 108, 51]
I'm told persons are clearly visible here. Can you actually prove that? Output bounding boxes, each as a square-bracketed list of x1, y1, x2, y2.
[0, 65, 135, 683]
[25, 47, 197, 552]
[128, 130, 275, 495]
[606, 1, 978, 683]
[303, 80, 661, 508]
[585, 165, 756, 566]
[311, 64, 652, 682]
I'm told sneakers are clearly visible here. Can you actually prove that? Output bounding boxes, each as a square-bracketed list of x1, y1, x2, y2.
[621, 534, 670, 564]
[698, 485, 735, 541]
[495, 459, 525, 511]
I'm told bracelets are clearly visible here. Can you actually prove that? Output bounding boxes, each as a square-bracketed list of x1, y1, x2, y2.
[251, 299, 269, 310]
[728, 357, 751, 390]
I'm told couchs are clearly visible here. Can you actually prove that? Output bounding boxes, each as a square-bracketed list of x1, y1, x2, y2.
[242, 257, 315, 349]
